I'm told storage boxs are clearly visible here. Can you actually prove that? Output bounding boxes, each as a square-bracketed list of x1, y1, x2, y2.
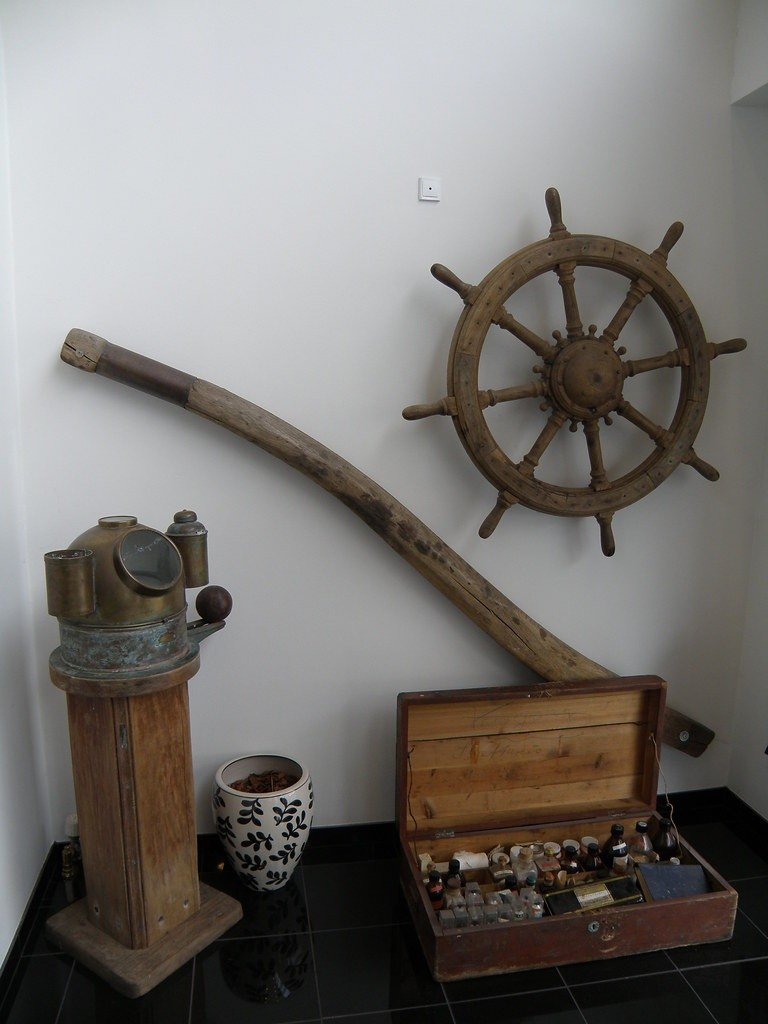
[392, 675, 739, 982]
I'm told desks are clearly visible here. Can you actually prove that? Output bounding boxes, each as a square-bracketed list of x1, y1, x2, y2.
[0, 786, 768, 1024]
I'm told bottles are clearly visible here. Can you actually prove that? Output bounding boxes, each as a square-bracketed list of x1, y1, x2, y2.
[426, 818, 673, 927]
